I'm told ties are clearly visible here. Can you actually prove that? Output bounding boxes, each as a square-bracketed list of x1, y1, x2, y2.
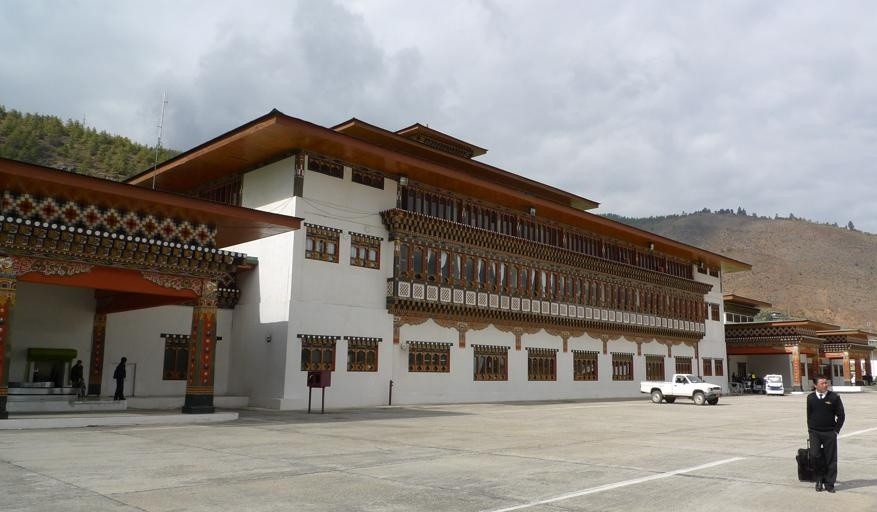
[819, 394, 823, 399]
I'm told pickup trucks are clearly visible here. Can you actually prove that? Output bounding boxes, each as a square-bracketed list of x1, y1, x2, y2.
[638, 373, 723, 404]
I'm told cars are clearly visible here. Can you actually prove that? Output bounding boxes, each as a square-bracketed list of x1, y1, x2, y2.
[849, 370, 877, 385]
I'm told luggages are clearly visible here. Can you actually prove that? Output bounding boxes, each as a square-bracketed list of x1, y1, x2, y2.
[796, 441, 823, 482]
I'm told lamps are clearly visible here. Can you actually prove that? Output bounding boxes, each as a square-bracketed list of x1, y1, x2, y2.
[649, 242, 654, 250]
[399, 176, 408, 186]
[529, 206, 536, 216]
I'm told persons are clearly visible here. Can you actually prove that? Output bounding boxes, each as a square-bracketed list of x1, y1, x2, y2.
[70, 360, 86, 399]
[806, 374, 845, 493]
[732, 372, 763, 388]
[113, 357, 127, 400]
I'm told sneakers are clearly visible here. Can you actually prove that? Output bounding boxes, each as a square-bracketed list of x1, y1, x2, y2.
[824, 485, 835, 493]
[816, 482, 822, 491]
